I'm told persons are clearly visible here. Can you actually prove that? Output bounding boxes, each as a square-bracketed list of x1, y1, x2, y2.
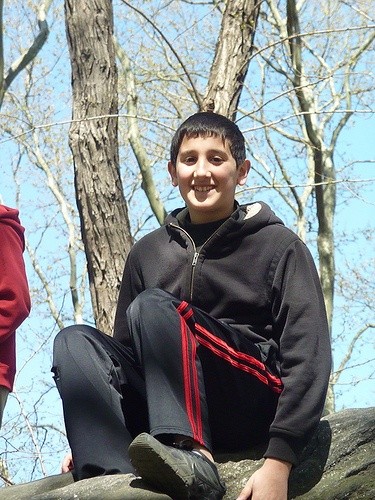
[0, 202, 30, 435]
[50, 112, 333, 500]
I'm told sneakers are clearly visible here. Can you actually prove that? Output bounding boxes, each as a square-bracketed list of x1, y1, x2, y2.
[128, 430, 226, 500]
